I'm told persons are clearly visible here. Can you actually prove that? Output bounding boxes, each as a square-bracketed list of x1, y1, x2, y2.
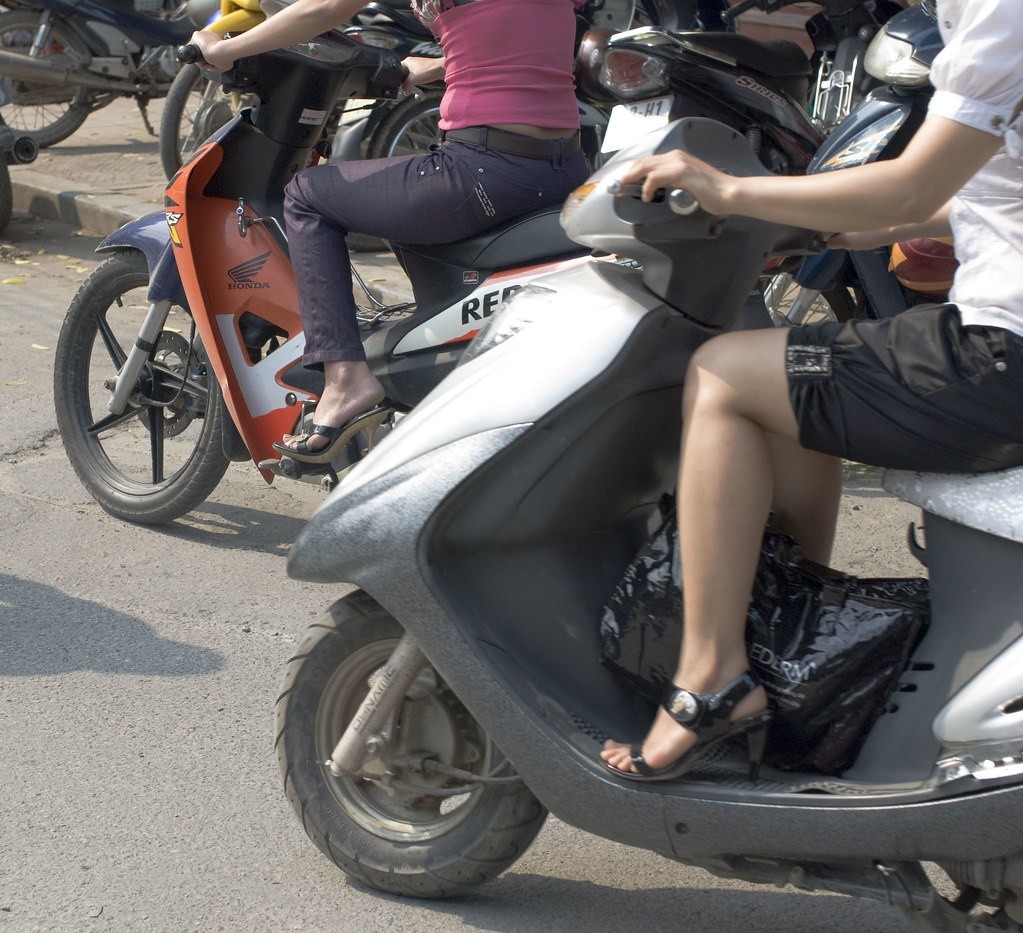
[600, 0, 1022, 782]
[188, 0, 586, 462]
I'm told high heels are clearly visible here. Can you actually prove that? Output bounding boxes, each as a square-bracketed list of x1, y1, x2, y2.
[599, 662, 772, 782]
[272, 407, 392, 464]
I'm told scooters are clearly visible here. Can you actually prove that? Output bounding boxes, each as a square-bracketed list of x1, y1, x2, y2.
[1, 1, 201, 237]
[55, 0, 1023, 933]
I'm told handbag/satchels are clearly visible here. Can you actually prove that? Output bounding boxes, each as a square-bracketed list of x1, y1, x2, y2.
[599, 501, 934, 777]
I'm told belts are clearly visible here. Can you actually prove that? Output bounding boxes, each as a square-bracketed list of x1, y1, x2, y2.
[443, 121, 582, 160]
